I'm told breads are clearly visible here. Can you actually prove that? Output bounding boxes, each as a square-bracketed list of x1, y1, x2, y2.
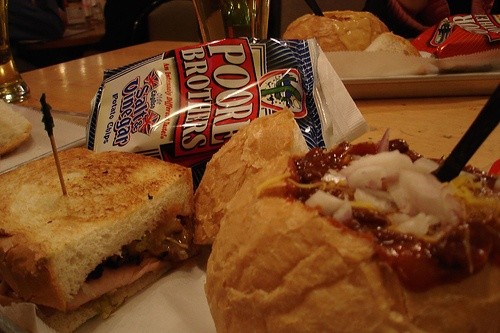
[283, 10, 387, 54]
[361, 30, 423, 58]
[203, 138, 500, 333]
[0, 98, 32, 157]
[188, 107, 308, 245]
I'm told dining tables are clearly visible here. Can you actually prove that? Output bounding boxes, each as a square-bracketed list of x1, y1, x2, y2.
[0, 40, 500, 333]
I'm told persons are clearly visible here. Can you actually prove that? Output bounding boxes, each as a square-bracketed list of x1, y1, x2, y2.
[9, 0, 67, 67]
[362, 0, 500, 38]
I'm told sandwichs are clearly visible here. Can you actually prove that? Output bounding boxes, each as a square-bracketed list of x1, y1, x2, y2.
[0, 146, 197, 333]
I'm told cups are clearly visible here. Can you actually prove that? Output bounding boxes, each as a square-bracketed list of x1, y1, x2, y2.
[0, 0, 32, 103]
[190, 0, 270, 42]
